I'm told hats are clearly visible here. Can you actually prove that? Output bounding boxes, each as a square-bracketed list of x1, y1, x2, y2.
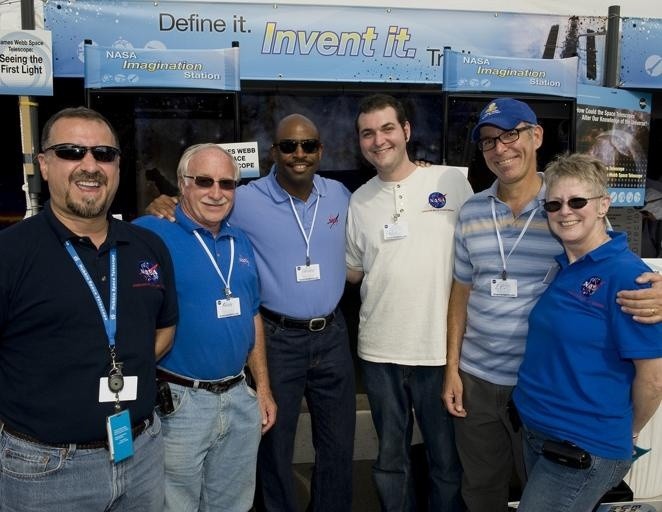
[471, 96, 538, 142]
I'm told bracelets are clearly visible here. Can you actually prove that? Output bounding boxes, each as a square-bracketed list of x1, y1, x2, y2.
[631, 435, 639, 439]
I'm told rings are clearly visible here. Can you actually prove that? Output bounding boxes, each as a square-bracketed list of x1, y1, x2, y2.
[651, 308, 657, 315]
[512, 149, 662, 512]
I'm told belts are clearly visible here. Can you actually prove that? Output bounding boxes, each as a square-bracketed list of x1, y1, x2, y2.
[258, 304, 342, 331]
[155, 367, 245, 395]
[1, 415, 153, 449]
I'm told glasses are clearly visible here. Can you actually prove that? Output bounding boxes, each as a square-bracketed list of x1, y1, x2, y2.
[271, 138, 322, 154]
[183, 175, 238, 190]
[42, 142, 121, 162]
[542, 195, 605, 213]
[476, 125, 534, 152]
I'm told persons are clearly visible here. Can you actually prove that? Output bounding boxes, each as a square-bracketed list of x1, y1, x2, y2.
[346, 93, 474, 512]
[129, 142, 278, 512]
[442, 96, 662, 511]
[145, 113, 360, 512]
[0, 106, 177, 512]
